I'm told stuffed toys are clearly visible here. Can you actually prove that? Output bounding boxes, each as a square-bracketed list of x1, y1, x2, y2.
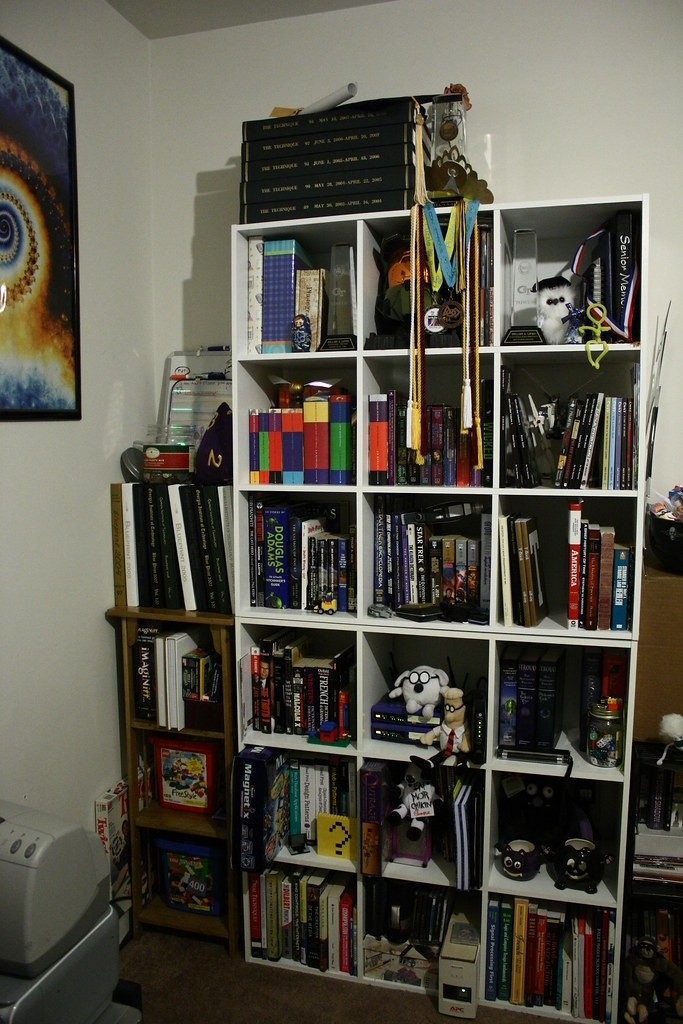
[408, 686, 474, 785]
[389, 664, 448, 718]
[533, 277, 575, 344]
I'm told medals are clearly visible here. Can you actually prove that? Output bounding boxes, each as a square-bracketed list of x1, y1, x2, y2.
[422, 301, 448, 337]
[438, 300, 464, 328]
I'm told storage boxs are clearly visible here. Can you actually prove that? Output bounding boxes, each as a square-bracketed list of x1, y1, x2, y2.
[631, 565, 683, 744]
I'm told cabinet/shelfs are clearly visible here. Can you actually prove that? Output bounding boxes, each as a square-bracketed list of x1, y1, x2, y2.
[228, 191, 652, 1024]
[106, 482, 241, 958]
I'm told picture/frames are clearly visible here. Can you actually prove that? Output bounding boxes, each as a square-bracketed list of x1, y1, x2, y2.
[0, 34, 82, 423]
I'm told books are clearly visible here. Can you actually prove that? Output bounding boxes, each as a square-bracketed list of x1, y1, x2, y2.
[245, 625, 356, 746]
[237, 91, 439, 216]
[514, 645, 540, 750]
[249, 492, 360, 624]
[375, 496, 489, 626]
[85, 726, 683, 1020]
[238, 225, 358, 354]
[128, 619, 160, 725]
[535, 645, 564, 752]
[497, 641, 514, 752]
[247, 365, 633, 493]
[133, 477, 235, 619]
[495, 496, 629, 635]
[149, 632, 168, 731]
[165, 624, 219, 731]
